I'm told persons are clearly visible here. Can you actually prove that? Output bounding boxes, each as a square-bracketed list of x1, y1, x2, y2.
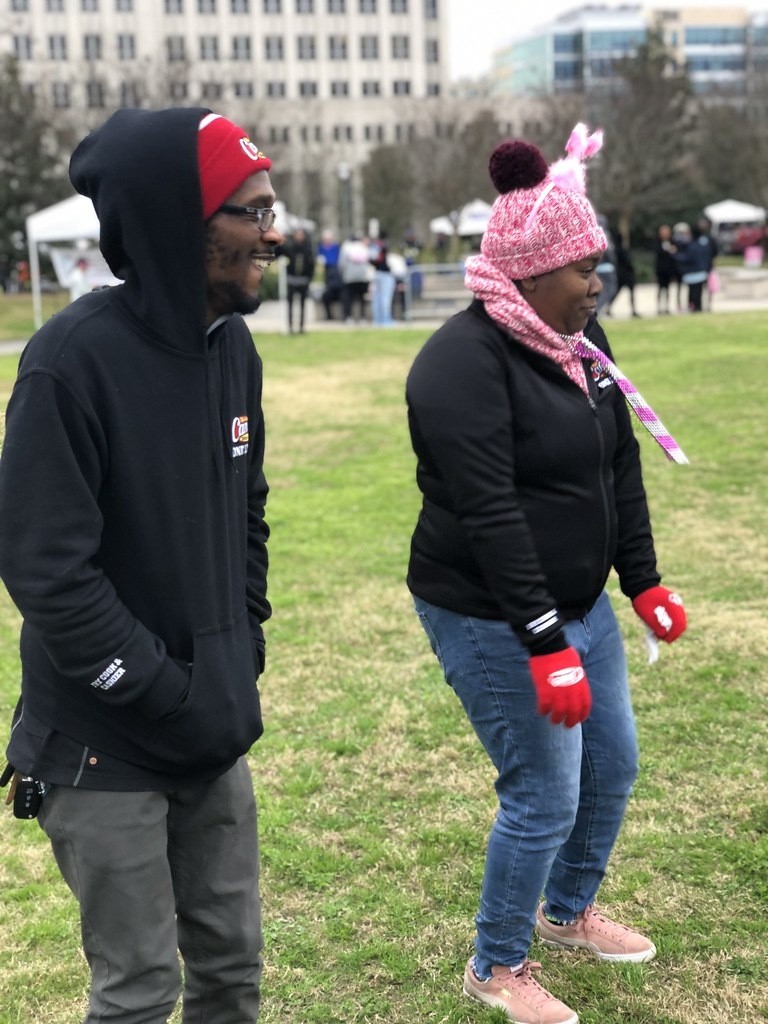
[402, 119, 690, 1024]
[278, 222, 411, 337]
[0, 104, 285, 1023]
[588, 209, 721, 320]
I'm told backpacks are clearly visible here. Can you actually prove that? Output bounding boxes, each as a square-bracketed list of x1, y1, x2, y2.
[387, 253, 406, 281]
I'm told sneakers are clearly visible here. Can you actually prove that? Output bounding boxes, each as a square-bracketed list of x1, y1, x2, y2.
[463, 896, 657, 1024]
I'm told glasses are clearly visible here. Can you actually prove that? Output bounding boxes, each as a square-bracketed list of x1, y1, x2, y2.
[221, 204, 276, 232]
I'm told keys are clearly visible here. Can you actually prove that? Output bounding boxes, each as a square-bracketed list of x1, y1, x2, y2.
[0, 762, 52, 819]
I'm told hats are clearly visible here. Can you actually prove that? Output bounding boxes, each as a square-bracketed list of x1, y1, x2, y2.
[197, 112, 273, 221]
[481, 122, 608, 277]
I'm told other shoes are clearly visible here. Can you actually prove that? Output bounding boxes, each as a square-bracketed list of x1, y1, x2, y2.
[606, 305, 713, 319]
[282, 313, 399, 336]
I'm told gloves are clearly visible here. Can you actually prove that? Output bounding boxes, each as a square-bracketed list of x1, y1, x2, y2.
[528, 585, 686, 727]
